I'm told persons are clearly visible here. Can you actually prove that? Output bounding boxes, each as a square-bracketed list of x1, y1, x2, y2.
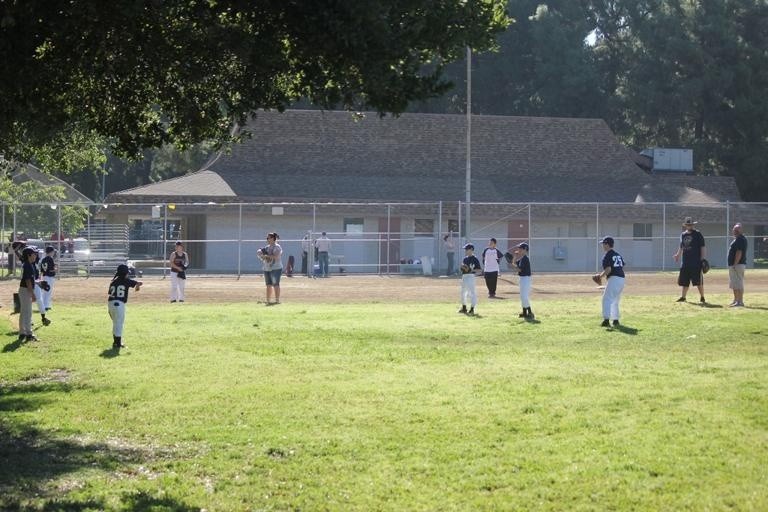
[482, 238, 503, 299]
[512, 242, 534, 319]
[107, 265, 142, 347]
[300, 236, 308, 274]
[444, 237, 456, 276]
[256, 233, 283, 304]
[673, 216, 708, 303]
[10, 229, 74, 341]
[169, 242, 189, 303]
[316, 232, 330, 275]
[727, 223, 748, 306]
[592, 236, 625, 326]
[459, 243, 481, 314]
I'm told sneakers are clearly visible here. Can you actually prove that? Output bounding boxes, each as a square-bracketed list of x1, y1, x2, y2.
[730, 300, 744, 306]
[699, 297, 705, 305]
[677, 297, 686, 302]
[42, 318, 51, 326]
[19, 335, 40, 342]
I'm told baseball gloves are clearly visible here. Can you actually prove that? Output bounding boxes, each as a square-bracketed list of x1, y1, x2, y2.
[504, 252, 513, 263]
[593, 275, 602, 285]
[37, 281, 50, 291]
[462, 263, 471, 273]
[257, 248, 269, 260]
[702, 259, 710, 273]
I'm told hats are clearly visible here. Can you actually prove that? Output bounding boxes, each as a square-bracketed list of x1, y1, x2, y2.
[683, 217, 698, 225]
[23, 248, 39, 256]
[600, 237, 614, 244]
[462, 243, 474, 251]
[516, 243, 529, 251]
[118, 264, 128, 272]
